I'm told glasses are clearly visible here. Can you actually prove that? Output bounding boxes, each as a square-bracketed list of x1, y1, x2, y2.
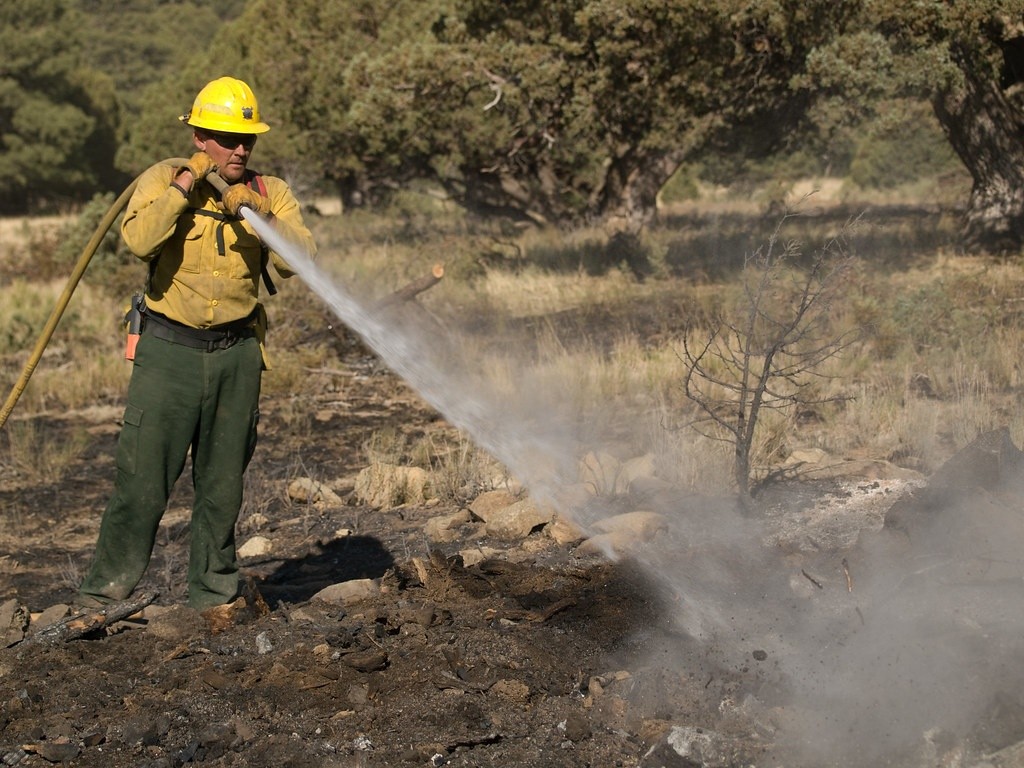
[201, 134, 258, 151]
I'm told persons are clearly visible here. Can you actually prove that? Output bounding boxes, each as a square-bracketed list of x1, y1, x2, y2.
[72, 77, 316, 614]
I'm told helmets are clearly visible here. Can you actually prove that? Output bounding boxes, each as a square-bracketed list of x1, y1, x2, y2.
[178, 77, 271, 134]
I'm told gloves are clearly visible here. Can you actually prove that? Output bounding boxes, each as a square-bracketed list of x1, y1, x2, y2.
[222, 183, 272, 217]
[174, 151, 217, 193]
